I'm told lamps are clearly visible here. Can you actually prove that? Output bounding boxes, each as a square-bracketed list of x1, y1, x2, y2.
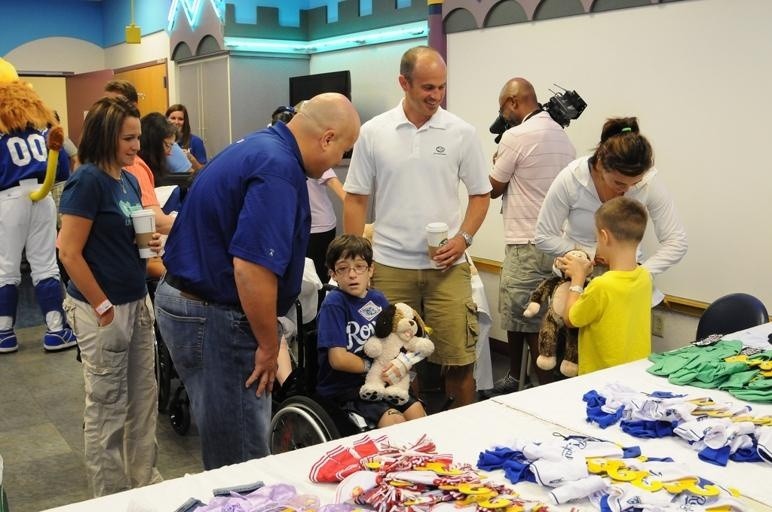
[126, 1, 142, 44]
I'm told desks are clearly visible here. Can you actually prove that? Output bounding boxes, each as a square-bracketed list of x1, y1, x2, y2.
[42, 401, 770, 511]
[493, 319, 770, 508]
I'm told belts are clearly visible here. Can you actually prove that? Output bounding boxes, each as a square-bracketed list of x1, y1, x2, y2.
[163, 273, 219, 306]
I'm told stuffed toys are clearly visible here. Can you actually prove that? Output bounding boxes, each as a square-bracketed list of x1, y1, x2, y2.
[523, 250, 594, 376]
[359, 303, 437, 405]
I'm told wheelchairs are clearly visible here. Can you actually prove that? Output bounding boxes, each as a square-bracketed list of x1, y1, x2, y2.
[153, 302, 193, 438]
[262, 283, 454, 458]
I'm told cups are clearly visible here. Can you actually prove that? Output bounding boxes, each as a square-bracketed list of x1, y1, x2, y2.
[424, 221, 449, 270]
[130, 208, 159, 259]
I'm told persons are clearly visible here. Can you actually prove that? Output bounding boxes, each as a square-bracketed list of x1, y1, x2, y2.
[342, 46, 494, 406]
[535, 118, 688, 307]
[314, 234, 425, 427]
[557, 197, 656, 376]
[1, 81, 207, 353]
[488, 80, 576, 392]
[153, 93, 361, 470]
[59, 97, 163, 498]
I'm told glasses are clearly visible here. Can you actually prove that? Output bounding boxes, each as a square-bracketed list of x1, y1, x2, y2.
[336, 265, 369, 274]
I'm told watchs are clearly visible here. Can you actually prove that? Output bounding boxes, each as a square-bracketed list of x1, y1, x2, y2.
[458, 230, 473, 246]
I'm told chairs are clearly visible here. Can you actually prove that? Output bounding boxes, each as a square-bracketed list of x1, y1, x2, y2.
[696, 292, 767, 344]
